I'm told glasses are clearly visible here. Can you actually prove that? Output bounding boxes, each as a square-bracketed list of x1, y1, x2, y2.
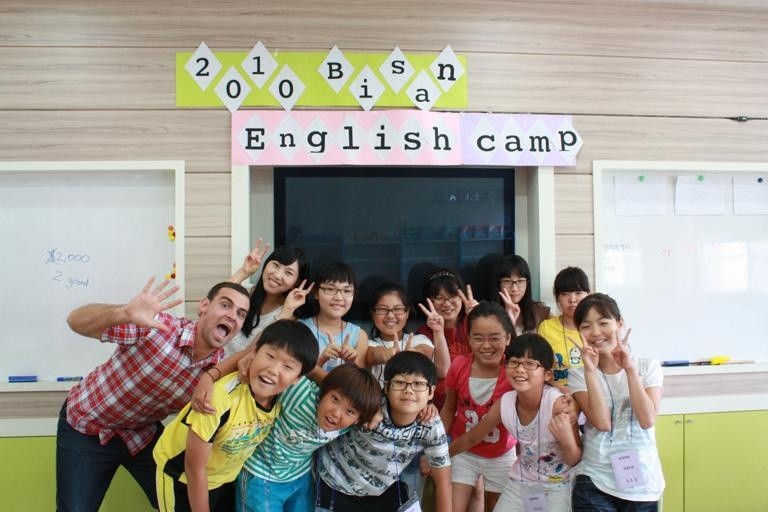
[373, 308, 405, 315]
[467, 336, 505, 344]
[504, 360, 541, 369]
[499, 280, 527, 286]
[384, 381, 429, 391]
[430, 295, 460, 304]
[320, 285, 355, 295]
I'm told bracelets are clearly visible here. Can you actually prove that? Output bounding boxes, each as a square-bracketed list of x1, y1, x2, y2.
[206, 367, 220, 382]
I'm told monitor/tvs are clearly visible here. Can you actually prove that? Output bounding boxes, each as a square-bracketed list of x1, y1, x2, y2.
[273, 167, 515, 322]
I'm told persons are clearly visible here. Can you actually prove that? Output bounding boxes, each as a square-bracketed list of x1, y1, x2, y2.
[306, 350, 453, 511]
[419, 332, 583, 511]
[536, 267, 589, 386]
[414, 265, 481, 411]
[153, 321, 319, 512]
[223, 238, 315, 362]
[295, 262, 369, 373]
[189, 348, 382, 512]
[364, 282, 435, 393]
[56, 275, 252, 512]
[494, 254, 557, 336]
[566, 292, 667, 512]
[438, 301, 517, 511]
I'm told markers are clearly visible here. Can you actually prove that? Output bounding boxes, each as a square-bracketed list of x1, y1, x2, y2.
[56, 377, 83, 381]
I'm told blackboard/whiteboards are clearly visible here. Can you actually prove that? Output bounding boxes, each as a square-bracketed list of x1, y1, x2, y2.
[0, 160, 185, 393]
[592, 159, 768, 377]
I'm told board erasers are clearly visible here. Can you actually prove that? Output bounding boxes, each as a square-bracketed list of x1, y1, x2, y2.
[9, 376, 37, 382]
[663, 360, 690, 366]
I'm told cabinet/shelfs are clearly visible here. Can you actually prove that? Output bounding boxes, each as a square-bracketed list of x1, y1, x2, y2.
[657, 411, 767, 512]
